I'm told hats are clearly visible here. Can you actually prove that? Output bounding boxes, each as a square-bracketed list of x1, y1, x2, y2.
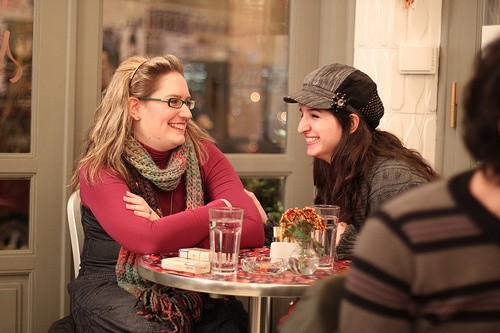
[283, 62, 384, 128]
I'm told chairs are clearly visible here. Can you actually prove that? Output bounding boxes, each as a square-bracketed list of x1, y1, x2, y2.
[67, 189, 85, 279]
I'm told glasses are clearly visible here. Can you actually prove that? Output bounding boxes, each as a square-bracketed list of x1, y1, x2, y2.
[138, 97, 196, 108]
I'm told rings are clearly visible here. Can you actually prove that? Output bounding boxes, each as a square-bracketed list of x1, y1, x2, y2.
[149, 209, 152, 214]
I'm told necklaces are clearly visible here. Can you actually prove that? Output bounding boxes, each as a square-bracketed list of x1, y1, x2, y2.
[169, 190, 173, 215]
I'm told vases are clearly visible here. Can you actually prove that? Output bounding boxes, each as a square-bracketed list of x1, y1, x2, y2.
[289, 239, 320, 276]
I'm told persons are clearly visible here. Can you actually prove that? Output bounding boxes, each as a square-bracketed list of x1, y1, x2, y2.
[331, 35, 499, 333]
[297, 63, 450, 263]
[68, 54, 265, 333]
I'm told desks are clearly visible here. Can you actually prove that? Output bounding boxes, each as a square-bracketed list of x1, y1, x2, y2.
[137, 248, 352, 333]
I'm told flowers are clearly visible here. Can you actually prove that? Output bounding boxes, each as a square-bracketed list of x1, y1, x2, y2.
[279, 206, 326, 268]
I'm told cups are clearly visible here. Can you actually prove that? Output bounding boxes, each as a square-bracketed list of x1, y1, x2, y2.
[273, 227, 289, 243]
[208, 207, 244, 276]
[270, 242, 300, 269]
[307, 204, 341, 269]
